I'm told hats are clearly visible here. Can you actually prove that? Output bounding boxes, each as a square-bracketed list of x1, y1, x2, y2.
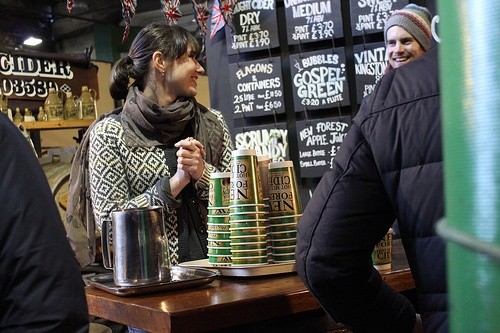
[383, 3, 434, 52]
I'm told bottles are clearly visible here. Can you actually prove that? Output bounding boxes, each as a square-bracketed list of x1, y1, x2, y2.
[24, 108, 35, 123]
[44, 88, 63, 123]
[37, 106, 47, 122]
[63, 91, 79, 120]
[12, 106, 24, 125]
[76, 87, 97, 120]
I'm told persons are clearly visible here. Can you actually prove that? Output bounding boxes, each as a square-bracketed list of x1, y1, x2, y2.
[383, 4, 433, 73]
[293, 31, 450, 333]
[0, 110, 90, 333]
[91, 23, 234, 333]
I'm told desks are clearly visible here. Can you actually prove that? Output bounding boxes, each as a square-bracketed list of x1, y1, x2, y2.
[83, 237, 416, 333]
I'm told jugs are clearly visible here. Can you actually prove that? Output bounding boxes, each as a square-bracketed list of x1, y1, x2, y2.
[101, 205, 172, 288]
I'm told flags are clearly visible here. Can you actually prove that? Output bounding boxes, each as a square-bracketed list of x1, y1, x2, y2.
[65, 0, 73, 14]
[120, 0, 137, 44]
[191, 0, 237, 40]
[160, 0, 182, 26]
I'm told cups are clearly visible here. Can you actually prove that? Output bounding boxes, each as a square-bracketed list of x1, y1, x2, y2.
[206, 149, 302, 269]
[371, 232, 393, 271]
